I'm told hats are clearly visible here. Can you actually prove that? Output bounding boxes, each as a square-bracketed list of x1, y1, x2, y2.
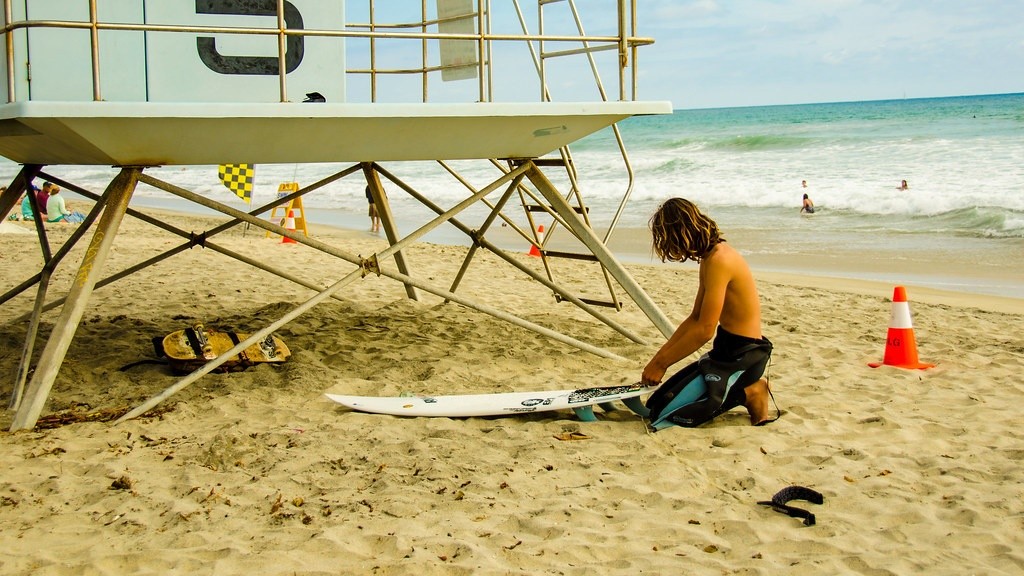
[32, 185, 41, 192]
[43, 181, 53, 186]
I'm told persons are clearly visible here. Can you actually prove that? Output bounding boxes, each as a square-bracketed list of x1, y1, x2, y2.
[642, 198, 774, 426]
[902, 180, 907, 189]
[800, 193, 815, 213]
[21, 181, 87, 223]
[801, 180, 808, 187]
[366, 185, 387, 232]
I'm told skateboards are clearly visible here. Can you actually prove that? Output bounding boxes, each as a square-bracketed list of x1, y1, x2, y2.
[160, 320, 292, 364]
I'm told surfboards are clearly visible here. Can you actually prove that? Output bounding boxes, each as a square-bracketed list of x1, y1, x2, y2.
[323, 379, 663, 419]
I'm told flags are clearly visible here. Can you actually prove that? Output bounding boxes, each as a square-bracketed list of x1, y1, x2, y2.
[218, 164, 254, 206]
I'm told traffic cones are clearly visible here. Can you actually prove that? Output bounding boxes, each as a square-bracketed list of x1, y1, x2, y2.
[525, 224, 545, 257]
[867, 285, 936, 371]
[276, 209, 297, 245]
